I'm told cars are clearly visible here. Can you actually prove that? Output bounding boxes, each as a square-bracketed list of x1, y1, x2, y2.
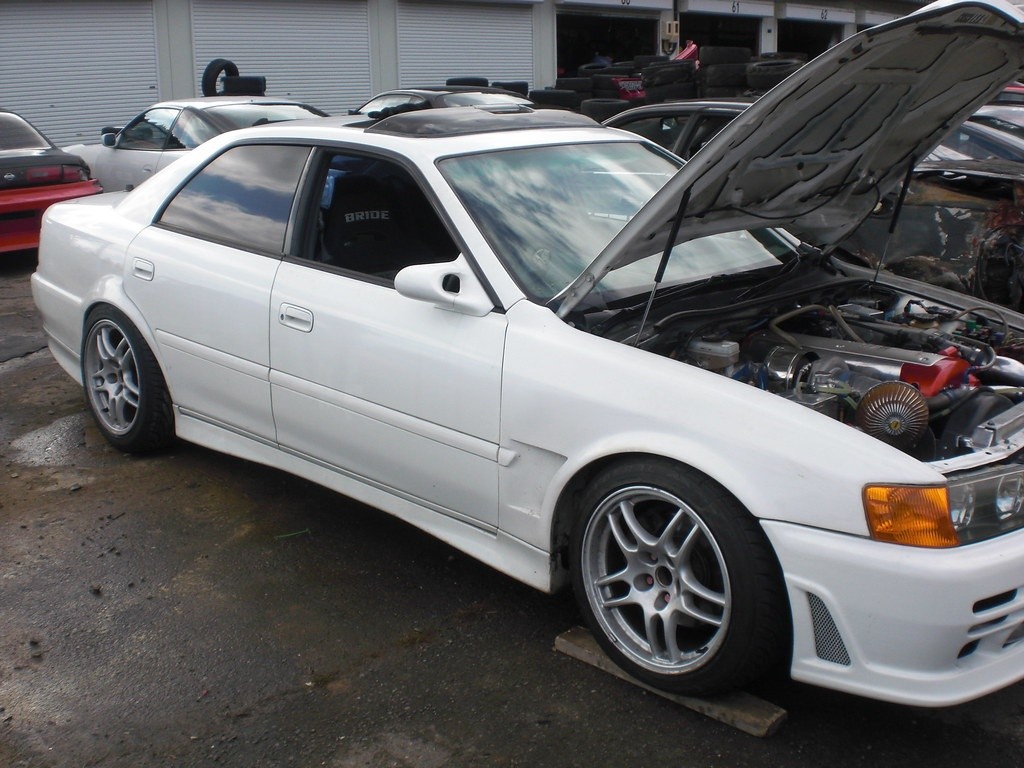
[1, 109, 105, 256]
[597, 60, 1024, 321]
[32, 1, 1024, 712]
[61, 95, 328, 192]
[347, 83, 541, 116]
